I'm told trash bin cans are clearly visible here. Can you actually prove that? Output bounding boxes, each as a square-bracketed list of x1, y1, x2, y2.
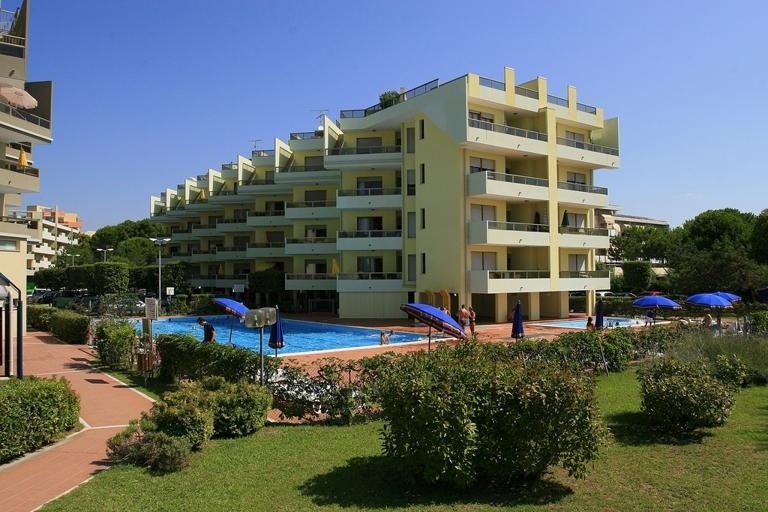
[137, 354, 152, 372]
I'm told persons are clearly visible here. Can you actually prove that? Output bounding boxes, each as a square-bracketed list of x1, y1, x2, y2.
[468, 306, 476, 336]
[442, 305, 451, 317]
[459, 303, 468, 332]
[196, 316, 217, 344]
[701, 307, 712, 325]
[586, 316, 596, 331]
[380, 331, 390, 346]
[596, 299, 604, 329]
[642, 307, 655, 327]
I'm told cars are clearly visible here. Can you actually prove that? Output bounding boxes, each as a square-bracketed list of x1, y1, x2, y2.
[27, 288, 145, 315]
[596, 291, 665, 297]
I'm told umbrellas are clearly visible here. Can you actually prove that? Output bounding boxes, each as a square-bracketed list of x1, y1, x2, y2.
[397, 302, 468, 355]
[712, 292, 742, 303]
[633, 294, 683, 323]
[212, 297, 249, 345]
[511, 299, 525, 342]
[686, 293, 735, 310]
[560, 209, 570, 228]
[0, 85, 39, 110]
[267, 305, 285, 359]
[17, 145, 29, 175]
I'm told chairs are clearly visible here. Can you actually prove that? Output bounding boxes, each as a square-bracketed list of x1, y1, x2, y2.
[683, 317, 756, 337]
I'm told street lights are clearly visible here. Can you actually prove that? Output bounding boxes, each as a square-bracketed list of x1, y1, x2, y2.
[67, 253, 80, 266]
[97, 248, 114, 262]
[149, 234, 171, 300]
